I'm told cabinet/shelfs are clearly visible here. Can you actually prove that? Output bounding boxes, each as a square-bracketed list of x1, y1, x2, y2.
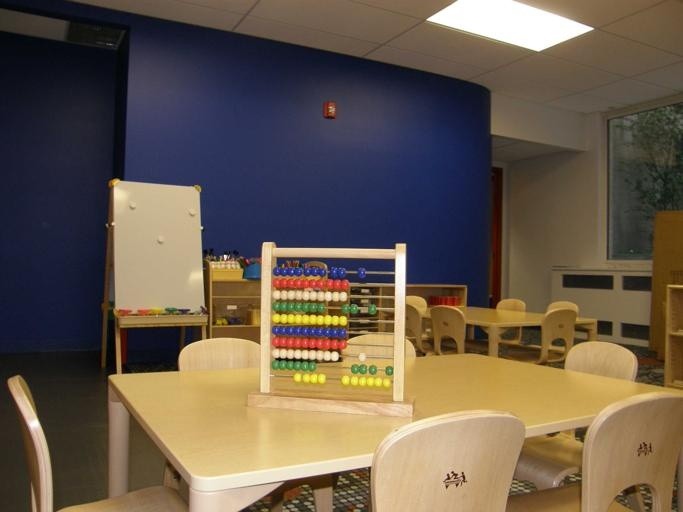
[665, 283, 683, 387]
[348, 283, 468, 341]
[204, 259, 327, 339]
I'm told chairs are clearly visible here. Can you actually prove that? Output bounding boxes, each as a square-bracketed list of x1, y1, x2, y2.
[562, 340, 637, 380]
[7, 370, 183, 511]
[506, 305, 581, 366]
[343, 330, 417, 362]
[423, 303, 468, 354]
[178, 339, 339, 511]
[530, 298, 579, 354]
[505, 390, 681, 511]
[371, 406, 528, 511]
[482, 296, 522, 347]
[406, 295, 434, 353]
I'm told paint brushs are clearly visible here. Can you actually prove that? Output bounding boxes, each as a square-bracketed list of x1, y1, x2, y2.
[203, 247, 239, 261]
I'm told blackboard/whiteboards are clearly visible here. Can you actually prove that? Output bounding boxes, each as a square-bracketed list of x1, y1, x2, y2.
[103, 179, 206, 315]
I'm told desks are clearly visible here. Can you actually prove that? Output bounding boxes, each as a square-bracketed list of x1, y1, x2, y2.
[418, 301, 600, 356]
[107, 350, 682, 512]
